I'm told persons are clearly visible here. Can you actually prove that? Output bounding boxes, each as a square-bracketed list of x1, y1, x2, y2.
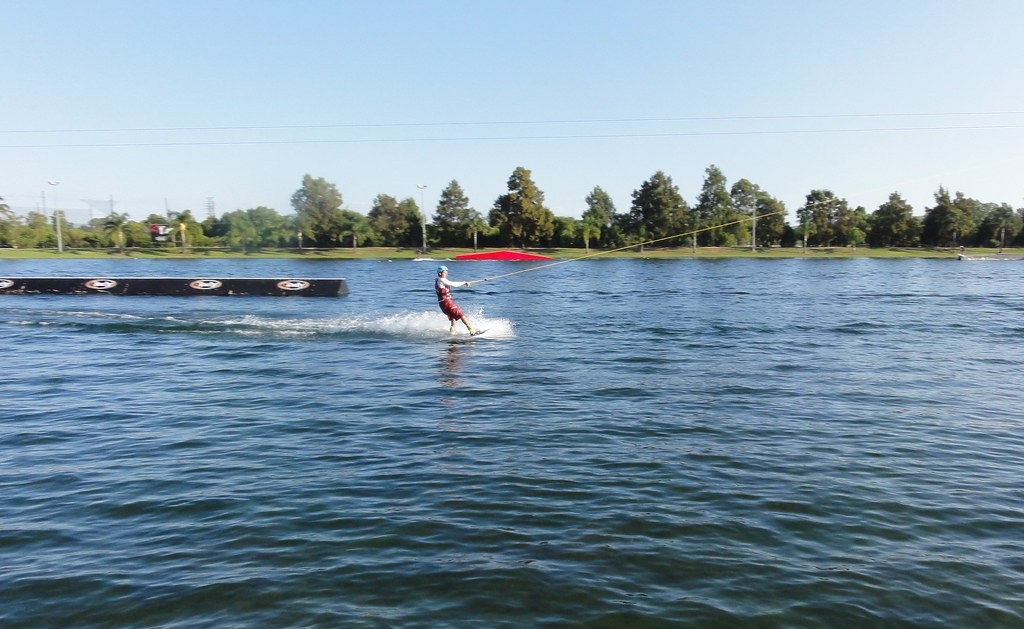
[435, 266, 479, 336]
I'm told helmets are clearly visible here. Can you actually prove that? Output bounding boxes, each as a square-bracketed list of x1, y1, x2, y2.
[437, 266, 448, 273]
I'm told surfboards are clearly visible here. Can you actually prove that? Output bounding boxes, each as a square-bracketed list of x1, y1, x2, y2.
[447, 329, 490, 336]
